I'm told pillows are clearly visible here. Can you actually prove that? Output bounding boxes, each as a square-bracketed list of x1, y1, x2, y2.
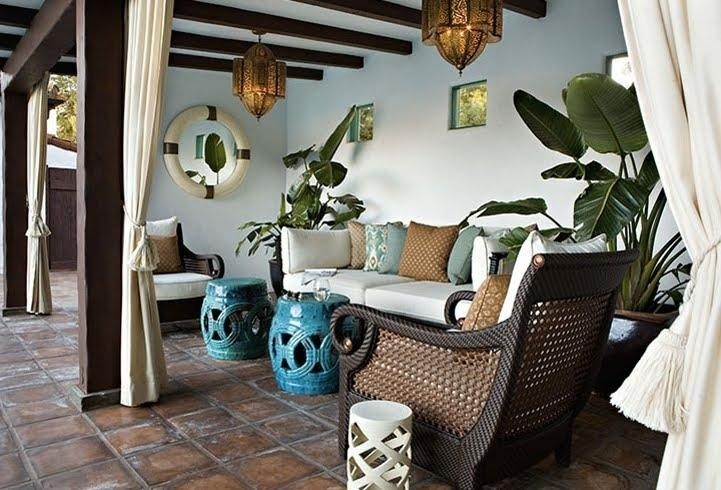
[148, 235, 180, 273]
[145, 217, 177, 236]
[281, 222, 509, 292]
[498, 231, 606, 324]
[461, 274, 511, 331]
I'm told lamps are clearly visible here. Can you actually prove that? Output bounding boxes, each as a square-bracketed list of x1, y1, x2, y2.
[422, 0, 503, 77]
[232, 27, 286, 121]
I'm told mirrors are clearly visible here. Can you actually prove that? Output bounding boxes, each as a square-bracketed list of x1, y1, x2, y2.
[163, 105, 252, 199]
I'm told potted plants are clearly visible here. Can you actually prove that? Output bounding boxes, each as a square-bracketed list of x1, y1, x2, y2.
[235, 104, 366, 296]
[456, 72, 692, 397]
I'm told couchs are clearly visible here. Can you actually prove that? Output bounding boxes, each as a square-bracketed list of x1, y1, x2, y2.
[275, 234, 509, 329]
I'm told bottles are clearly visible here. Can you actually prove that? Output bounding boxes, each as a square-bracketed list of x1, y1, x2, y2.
[312, 277, 331, 303]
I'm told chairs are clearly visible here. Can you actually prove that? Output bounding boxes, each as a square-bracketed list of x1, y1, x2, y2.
[145, 223, 225, 323]
[329, 249, 639, 489]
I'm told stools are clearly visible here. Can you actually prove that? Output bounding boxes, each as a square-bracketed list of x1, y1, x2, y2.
[200, 277, 274, 361]
[268, 292, 350, 395]
[347, 401, 413, 489]
